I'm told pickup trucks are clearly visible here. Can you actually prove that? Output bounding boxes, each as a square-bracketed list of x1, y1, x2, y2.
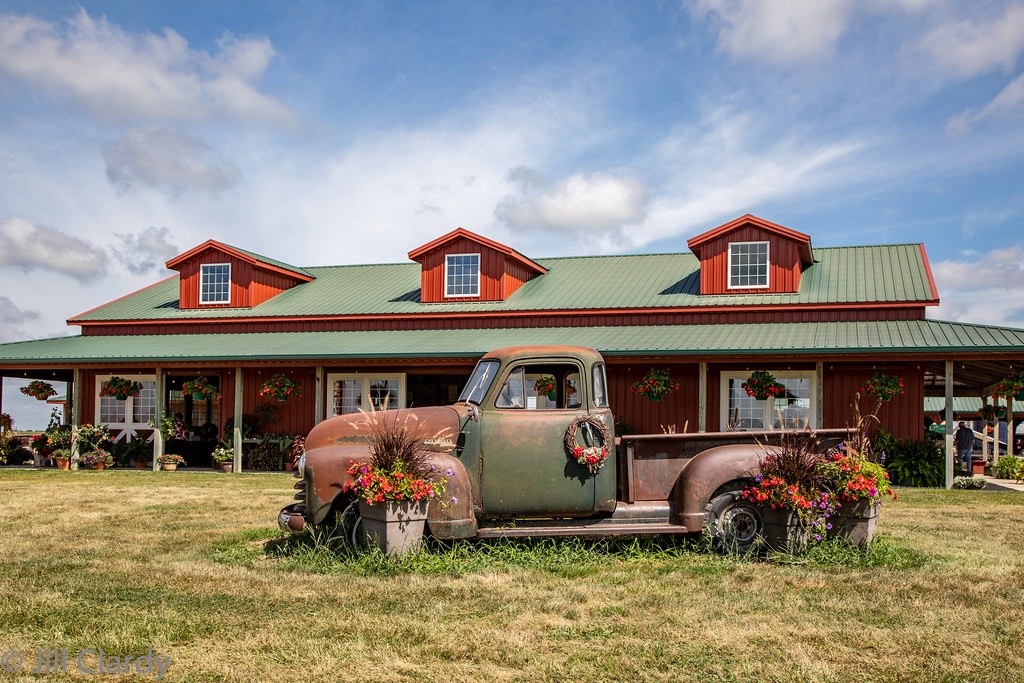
[274, 347, 837, 559]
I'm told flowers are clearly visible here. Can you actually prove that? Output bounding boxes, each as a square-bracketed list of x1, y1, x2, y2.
[992, 372, 1024, 400]
[18, 381, 59, 397]
[858, 376, 906, 397]
[939, 408, 960, 419]
[977, 404, 993, 417]
[994, 405, 1010, 412]
[629, 367, 679, 398]
[741, 371, 785, 397]
[740, 429, 903, 519]
[533, 376, 575, 398]
[344, 421, 464, 500]
[260, 373, 305, 398]
[99, 377, 146, 398]
[35, 412, 184, 462]
[184, 376, 222, 399]
[210, 447, 298, 462]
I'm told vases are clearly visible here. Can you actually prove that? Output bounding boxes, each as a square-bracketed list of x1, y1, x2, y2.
[277, 392, 288, 401]
[875, 389, 895, 400]
[996, 411, 1006, 418]
[942, 417, 957, 421]
[218, 459, 295, 473]
[190, 392, 205, 400]
[1013, 389, 1024, 401]
[357, 495, 433, 554]
[648, 389, 663, 399]
[36, 390, 48, 401]
[984, 412, 993, 421]
[54, 456, 178, 470]
[548, 388, 560, 401]
[756, 391, 769, 401]
[115, 392, 131, 400]
[763, 492, 876, 554]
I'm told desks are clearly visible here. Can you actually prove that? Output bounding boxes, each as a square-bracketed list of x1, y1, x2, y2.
[219, 438, 293, 471]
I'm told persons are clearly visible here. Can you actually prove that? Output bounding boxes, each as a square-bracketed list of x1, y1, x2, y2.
[171, 412, 189, 440]
[955, 422, 974, 473]
